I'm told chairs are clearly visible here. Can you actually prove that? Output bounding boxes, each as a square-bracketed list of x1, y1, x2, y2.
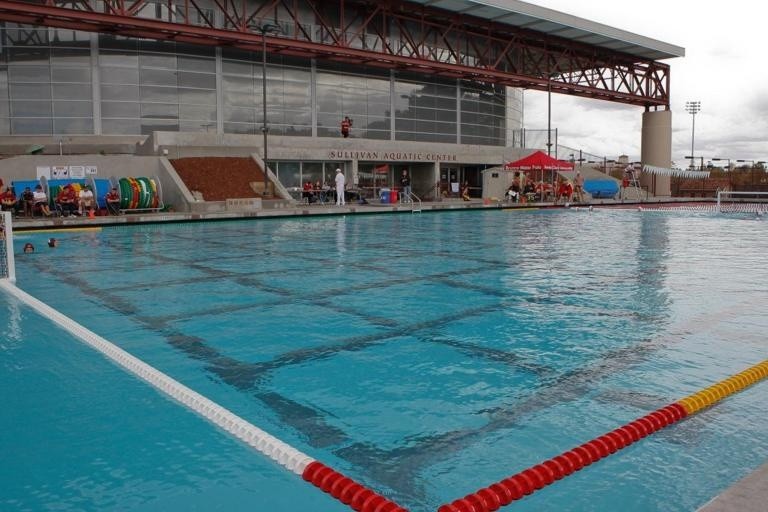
[2, 197, 121, 217]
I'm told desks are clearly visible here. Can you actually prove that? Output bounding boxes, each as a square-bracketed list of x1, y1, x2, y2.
[300, 189, 335, 203]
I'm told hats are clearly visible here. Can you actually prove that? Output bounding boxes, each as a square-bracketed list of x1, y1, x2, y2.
[48, 238, 56, 247]
[24, 243, 34, 252]
[336, 168, 341, 172]
[7, 184, 118, 191]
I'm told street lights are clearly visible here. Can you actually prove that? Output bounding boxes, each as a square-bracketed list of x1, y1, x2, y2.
[245, 22, 281, 191]
[684, 101, 701, 170]
[569, 157, 616, 176]
[758, 161, 768, 164]
[735, 160, 754, 190]
[684, 156, 704, 193]
[712, 158, 731, 197]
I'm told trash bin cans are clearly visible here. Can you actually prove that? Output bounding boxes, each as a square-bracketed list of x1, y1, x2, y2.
[390, 191, 397, 203]
[380, 189, 391, 203]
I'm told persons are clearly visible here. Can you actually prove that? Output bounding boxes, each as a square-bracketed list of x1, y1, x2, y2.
[24, 237, 58, 253]
[626, 162, 639, 180]
[302, 168, 346, 206]
[400, 169, 411, 203]
[340, 116, 351, 138]
[508, 170, 585, 202]
[0, 179, 121, 218]
